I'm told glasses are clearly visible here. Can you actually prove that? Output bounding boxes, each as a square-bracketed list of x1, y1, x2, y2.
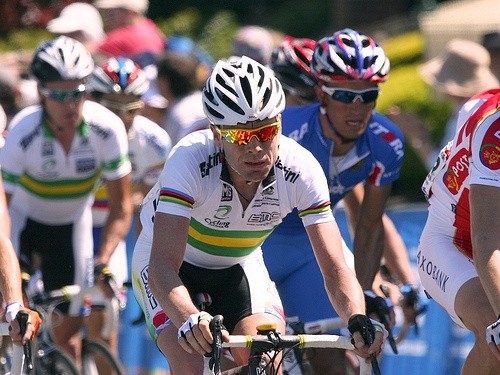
[214, 114, 281, 145]
[100, 100, 144, 115]
[40, 83, 85, 102]
[281, 81, 316, 104]
[321, 85, 381, 104]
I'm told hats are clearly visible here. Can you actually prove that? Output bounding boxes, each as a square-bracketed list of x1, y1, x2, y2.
[418, 39, 500, 97]
[46, 2, 104, 41]
[94, 0, 149, 14]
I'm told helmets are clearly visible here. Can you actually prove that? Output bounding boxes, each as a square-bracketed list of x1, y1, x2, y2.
[311, 28, 390, 83]
[85, 57, 149, 96]
[271, 37, 317, 87]
[202, 56, 285, 125]
[31, 35, 94, 81]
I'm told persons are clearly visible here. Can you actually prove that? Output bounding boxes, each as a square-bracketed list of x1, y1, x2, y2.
[131, 54, 389, 375]
[0, 36, 135, 375]
[417, 86, 500, 375]
[1, 175, 42, 346]
[81, 56, 171, 374]
[382, 36, 500, 174]
[0, 0, 430, 340]
[261, 28, 404, 375]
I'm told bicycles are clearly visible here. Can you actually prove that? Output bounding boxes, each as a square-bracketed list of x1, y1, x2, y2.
[0, 273, 435, 375]
